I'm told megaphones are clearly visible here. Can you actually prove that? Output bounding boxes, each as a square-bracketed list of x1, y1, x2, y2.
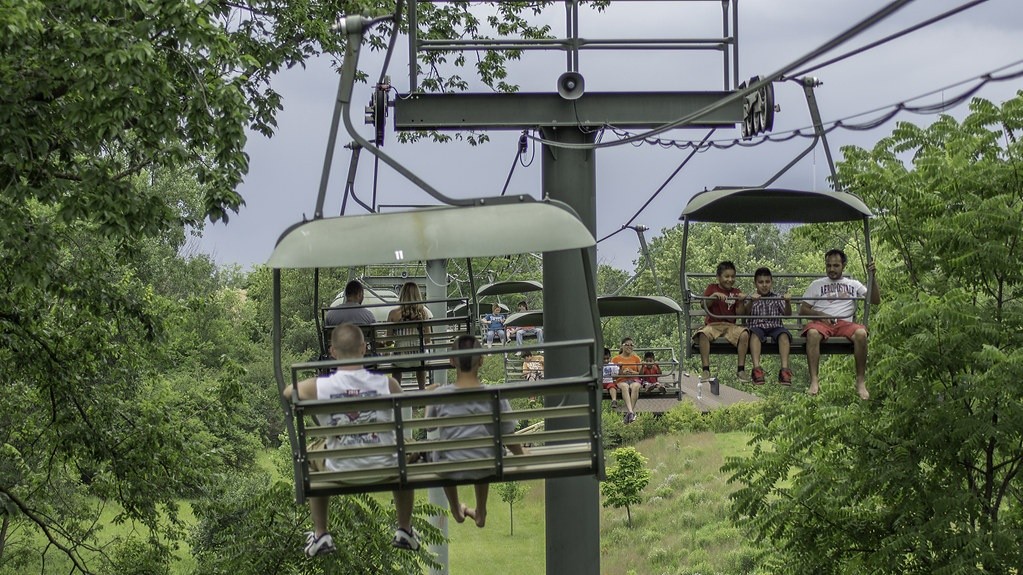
[557, 72, 585, 99]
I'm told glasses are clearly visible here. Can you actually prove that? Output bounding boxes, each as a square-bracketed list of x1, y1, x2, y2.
[623, 343, 634, 346]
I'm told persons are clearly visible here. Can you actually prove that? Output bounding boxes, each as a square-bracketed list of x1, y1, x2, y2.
[692, 261, 750, 383]
[480, 301, 545, 356]
[386, 282, 434, 390]
[799, 249, 880, 400]
[424, 336, 530, 528]
[743, 268, 793, 385]
[282, 323, 422, 557]
[326, 281, 379, 371]
[603, 338, 667, 426]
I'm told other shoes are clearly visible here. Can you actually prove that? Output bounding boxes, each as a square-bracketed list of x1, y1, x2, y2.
[612, 400, 617, 408]
[735, 371, 750, 382]
[779, 368, 794, 386]
[645, 384, 654, 395]
[623, 410, 636, 424]
[658, 386, 668, 395]
[530, 397, 536, 403]
[751, 367, 765, 385]
[699, 370, 711, 383]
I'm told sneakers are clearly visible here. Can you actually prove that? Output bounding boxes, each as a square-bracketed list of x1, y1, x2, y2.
[392, 525, 421, 551]
[304, 532, 337, 558]
